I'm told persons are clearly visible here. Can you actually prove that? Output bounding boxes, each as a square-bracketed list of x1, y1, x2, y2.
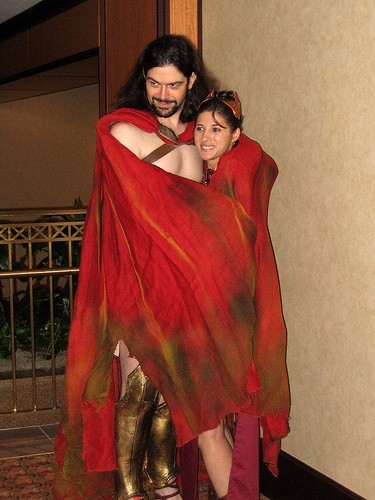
[54, 34, 292, 500]
